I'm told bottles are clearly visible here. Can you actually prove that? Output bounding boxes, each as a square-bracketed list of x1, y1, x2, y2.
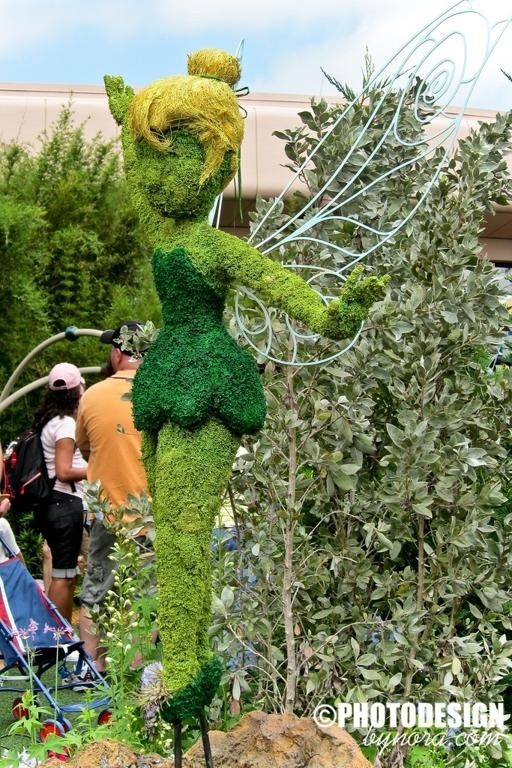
[48, 362, 86, 391]
[100, 321, 149, 354]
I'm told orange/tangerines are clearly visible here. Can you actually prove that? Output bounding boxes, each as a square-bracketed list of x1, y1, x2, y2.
[0, 517, 121, 763]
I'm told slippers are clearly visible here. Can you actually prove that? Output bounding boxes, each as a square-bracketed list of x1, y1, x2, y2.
[5, 410, 78, 513]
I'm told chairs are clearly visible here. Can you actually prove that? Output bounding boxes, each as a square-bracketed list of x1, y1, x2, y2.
[62, 672, 104, 694]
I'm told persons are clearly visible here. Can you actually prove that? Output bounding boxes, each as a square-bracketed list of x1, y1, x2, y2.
[0, 320, 271, 742]
